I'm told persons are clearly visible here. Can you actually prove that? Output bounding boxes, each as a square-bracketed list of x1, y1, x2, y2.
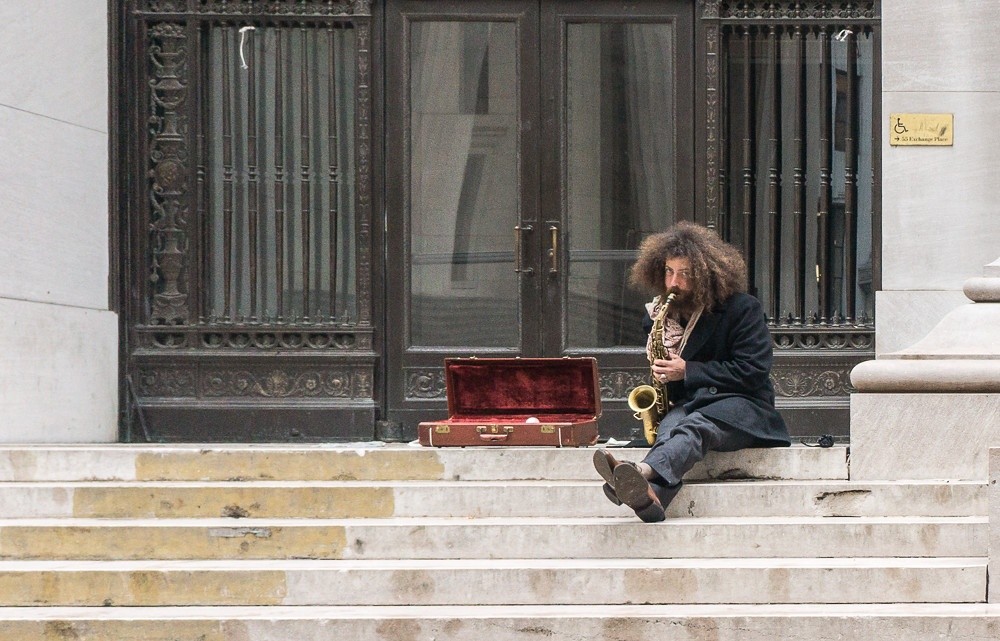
[593, 220, 789, 522]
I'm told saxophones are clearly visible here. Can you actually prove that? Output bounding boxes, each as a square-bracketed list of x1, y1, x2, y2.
[628, 293, 676, 446]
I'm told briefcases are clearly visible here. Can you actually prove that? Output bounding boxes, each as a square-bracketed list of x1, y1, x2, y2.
[416, 355, 605, 447]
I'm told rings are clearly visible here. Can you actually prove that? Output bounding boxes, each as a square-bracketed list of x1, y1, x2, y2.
[661, 373, 667, 379]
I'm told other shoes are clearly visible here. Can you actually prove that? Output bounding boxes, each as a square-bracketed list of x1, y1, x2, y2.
[591, 447, 638, 507]
[612, 462, 666, 524]
